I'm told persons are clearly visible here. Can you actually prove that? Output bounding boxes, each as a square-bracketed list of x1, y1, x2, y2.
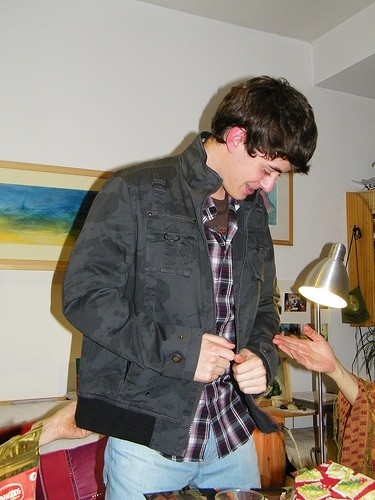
[61, 76, 319, 500]
[272, 324, 375, 480]
[0, 399, 95, 448]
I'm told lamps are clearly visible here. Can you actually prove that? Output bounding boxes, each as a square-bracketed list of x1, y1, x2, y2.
[299, 243, 349, 466]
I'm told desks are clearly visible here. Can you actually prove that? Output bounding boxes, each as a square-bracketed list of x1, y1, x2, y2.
[143, 488, 375, 500]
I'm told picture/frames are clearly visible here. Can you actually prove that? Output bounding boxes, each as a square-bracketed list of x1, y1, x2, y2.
[0, 160, 117, 270]
[261, 170, 293, 246]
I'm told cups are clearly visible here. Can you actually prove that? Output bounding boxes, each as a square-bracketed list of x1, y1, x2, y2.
[215, 489, 265, 500]
